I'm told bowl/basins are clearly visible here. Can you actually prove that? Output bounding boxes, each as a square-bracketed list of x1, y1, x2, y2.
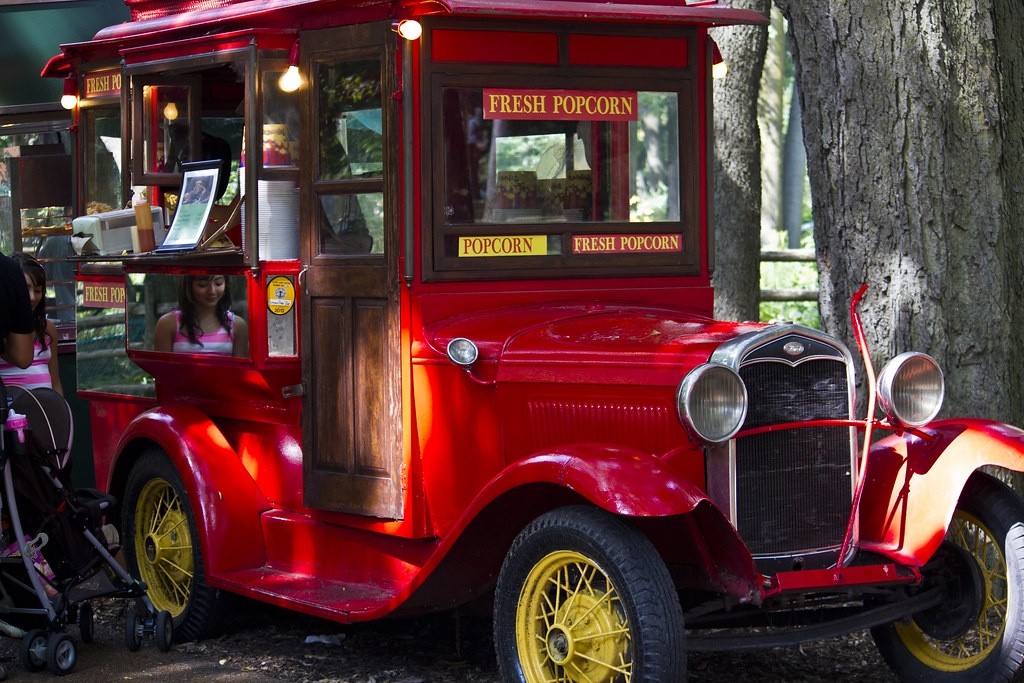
[238, 122, 299, 260]
[491, 168, 592, 222]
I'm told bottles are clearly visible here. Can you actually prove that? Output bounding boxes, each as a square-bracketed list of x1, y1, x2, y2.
[134, 192, 156, 252]
[6, 408, 28, 443]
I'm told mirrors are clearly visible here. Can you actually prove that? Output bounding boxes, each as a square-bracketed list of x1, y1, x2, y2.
[76, 273, 251, 397]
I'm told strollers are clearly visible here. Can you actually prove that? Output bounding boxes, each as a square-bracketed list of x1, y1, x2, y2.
[0, 381, 175, 676]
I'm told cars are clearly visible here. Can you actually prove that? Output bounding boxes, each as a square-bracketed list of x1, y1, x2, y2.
[38, 1, 1024, 683]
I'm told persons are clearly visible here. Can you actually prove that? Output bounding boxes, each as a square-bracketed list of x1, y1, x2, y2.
[286, 96, 374, 255]
[153, 272, 249, 358]
[0, 251, 63, 443]
[0, 251, 35, 424]
